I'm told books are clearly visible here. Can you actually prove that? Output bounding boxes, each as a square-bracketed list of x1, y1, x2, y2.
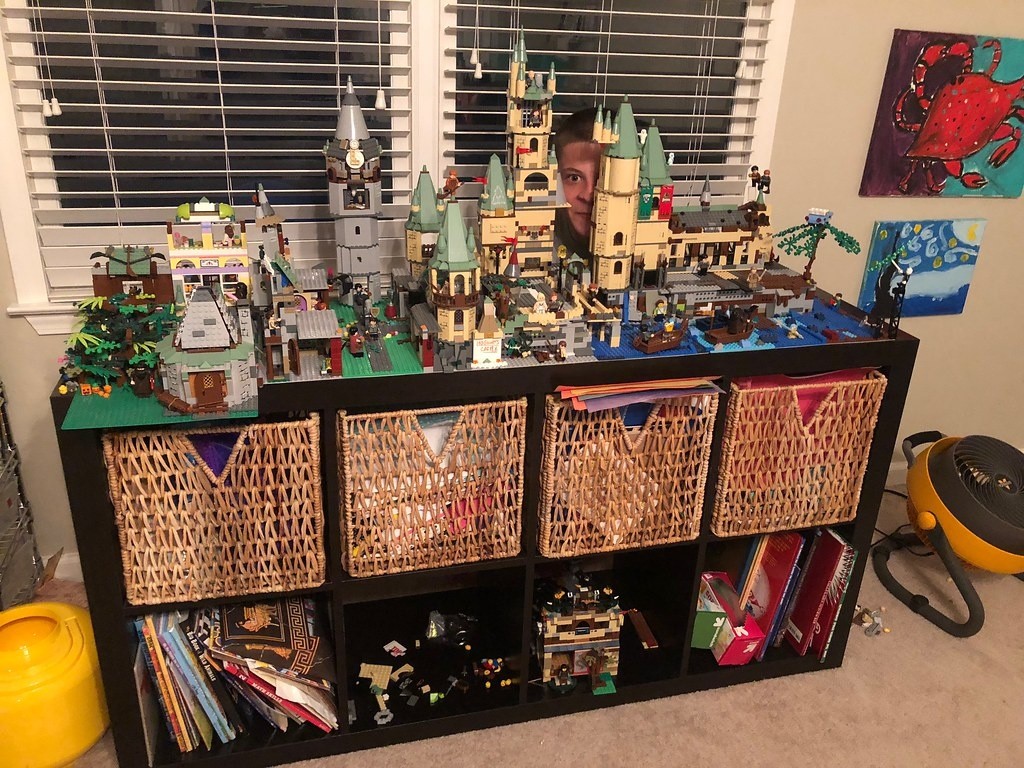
[735, 527, 858, 663]
[133, 598, 339, 753]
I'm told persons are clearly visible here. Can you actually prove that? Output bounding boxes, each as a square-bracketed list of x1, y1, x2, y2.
[349, 317, 381, 354]
[266, 311, 281, 336]
[354, 283, 368, 301]
[832, 293, 843, 307]
[222, 225, 234, 246]
[442, 170, 462, 201]
[897, 267, 913, 288]
[697, 254, 710, 276]
[588, 284, 597, 306]
[749, 166, 771, 194]
[552, 107, 616, 265]
[439, 277, 450, 295]
[532, 292, 560, 313]
[559, 341, 566, 361]
[654, 300, 666, 322]
[747, 266, 762, 288]
[528, 110, 543, 128]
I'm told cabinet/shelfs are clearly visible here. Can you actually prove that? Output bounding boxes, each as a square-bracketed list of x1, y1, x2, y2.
[48, 282, 921, 768]
